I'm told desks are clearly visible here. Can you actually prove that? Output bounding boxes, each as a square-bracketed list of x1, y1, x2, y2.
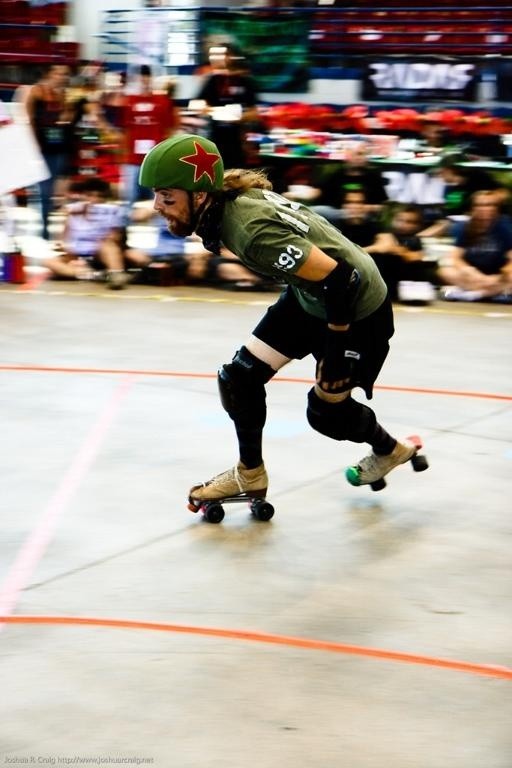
[256, 127, 468, 210]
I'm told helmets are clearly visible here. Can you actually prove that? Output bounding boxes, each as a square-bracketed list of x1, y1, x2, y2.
[135, 132, 226, 194]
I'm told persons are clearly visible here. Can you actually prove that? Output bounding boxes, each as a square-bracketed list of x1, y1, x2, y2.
[137, 132, 418, 500]
[2, 40, 512, 305]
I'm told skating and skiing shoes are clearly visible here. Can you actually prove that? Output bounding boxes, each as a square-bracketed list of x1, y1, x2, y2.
[344, 431, 432, 493]
[190, 461, 280, 531]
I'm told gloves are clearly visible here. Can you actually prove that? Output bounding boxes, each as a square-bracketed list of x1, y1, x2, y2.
[320, 353, 351, 384]
